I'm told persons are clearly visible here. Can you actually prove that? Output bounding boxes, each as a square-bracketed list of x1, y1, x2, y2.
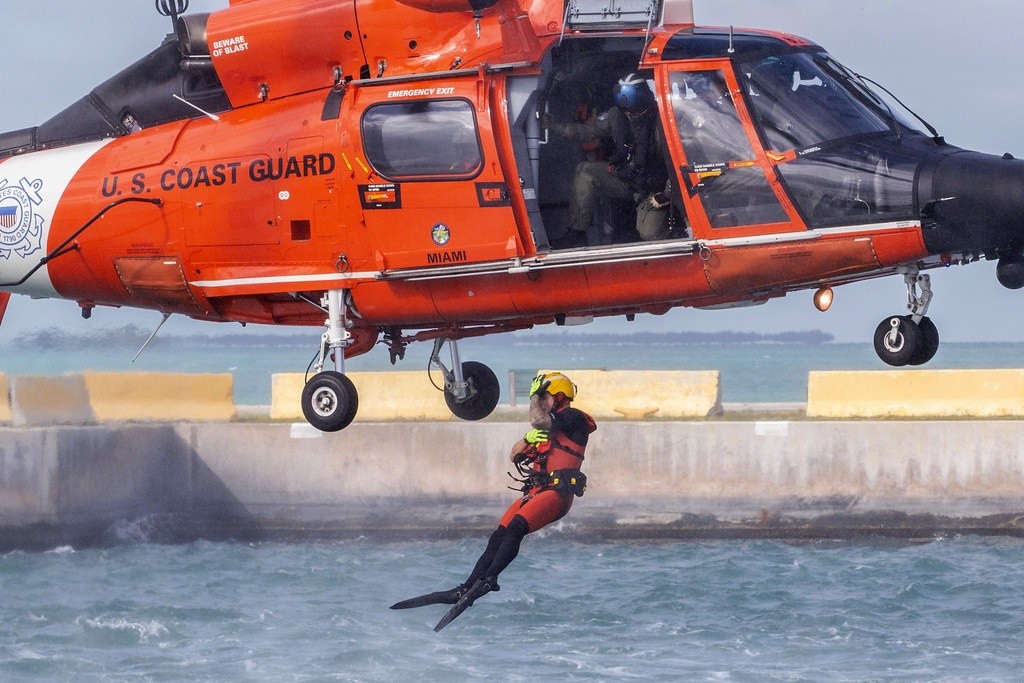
[542, 73, 689, 250]
[678, 55, 861, 218]
[389, 372, 597, 633]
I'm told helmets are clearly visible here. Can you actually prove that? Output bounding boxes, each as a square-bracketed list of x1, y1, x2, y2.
[540, 372, 577, 398]
[612, 73, 649, 111]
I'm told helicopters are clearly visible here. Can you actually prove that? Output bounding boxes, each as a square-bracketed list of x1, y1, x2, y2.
[0, 0, 1024, 434]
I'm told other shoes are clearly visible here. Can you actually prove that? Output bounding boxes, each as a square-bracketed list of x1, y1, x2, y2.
[551, 230, 589, 249]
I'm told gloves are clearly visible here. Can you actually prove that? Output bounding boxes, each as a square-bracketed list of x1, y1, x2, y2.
[529, 374, 551, 399]
[524, 428, 549, 444]
[541, 112, 560, 134]
